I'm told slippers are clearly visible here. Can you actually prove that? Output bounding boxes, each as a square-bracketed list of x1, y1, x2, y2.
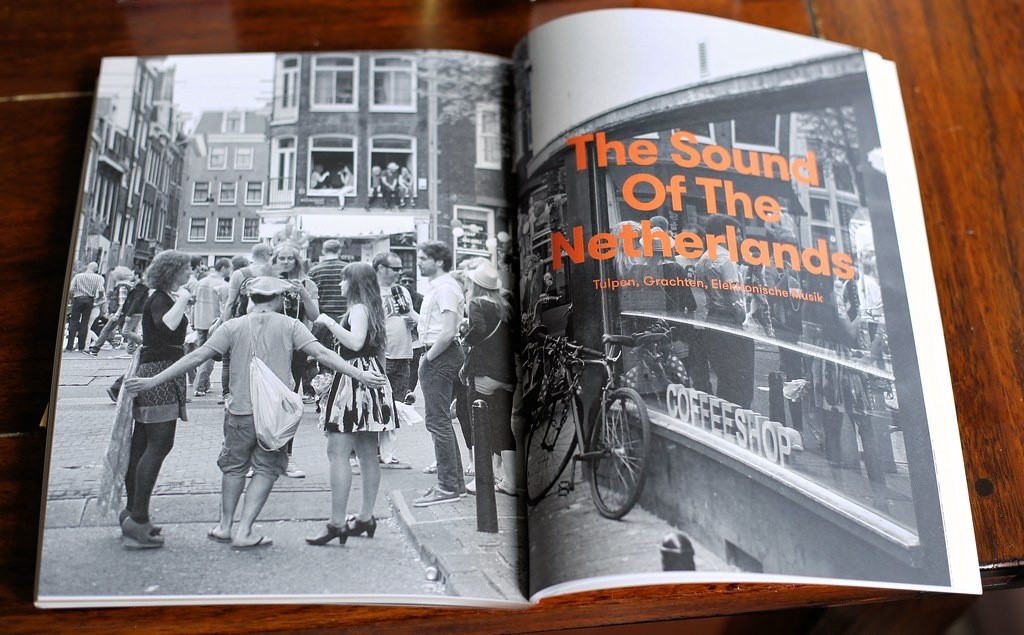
[494, 484, 516, 496]
[230, 534, 273, 550]
[208, 526, 231, 543]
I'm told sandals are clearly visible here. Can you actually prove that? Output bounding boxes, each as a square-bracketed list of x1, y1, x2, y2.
[423, 465, 438, 474]
[465, 467, 475, 476]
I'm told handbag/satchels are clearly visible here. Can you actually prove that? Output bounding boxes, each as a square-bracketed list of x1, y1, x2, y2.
[91, 316, 109, 337]
[249, 355, 304, 451]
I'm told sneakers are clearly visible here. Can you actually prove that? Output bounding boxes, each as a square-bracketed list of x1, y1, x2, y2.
[411, 484, 461, 506]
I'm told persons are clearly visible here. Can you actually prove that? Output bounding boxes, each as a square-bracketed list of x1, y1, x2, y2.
[407, 239, 468, 509]
[123, 275, 386, 549]
[304, 260, 389, 547]
[65, 160, 558, 494]
[118, 249, 199, 548]
[611, 207, 906, 484]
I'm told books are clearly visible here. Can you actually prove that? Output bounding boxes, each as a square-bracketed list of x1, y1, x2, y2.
[29, 9, 985, 613]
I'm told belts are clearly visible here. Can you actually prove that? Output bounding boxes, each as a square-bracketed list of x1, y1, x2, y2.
[80, 296, 90, 299]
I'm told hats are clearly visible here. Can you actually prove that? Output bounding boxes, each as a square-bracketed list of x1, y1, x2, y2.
[465, 258, 502, 290]
[246, 276, 292, 295]
[640, 227, 676, 252]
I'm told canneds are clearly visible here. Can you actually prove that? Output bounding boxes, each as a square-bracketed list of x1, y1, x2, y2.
[390, 284, 410, 314]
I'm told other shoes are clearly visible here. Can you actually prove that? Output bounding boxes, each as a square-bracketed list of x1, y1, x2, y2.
[404, 391, 416, 406]
[188, 379, 228, 404]
[350, 459, 361, 474]
[379, 455, 412, 469]
[284, 457, 306, 478]
[62, 337, 143, 358]
[493, 474, 503, 483]
[107, 384, 117, 402]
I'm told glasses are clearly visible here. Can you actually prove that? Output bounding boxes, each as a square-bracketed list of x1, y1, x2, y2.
[401, 280, 414, 284]
[383, 265, 406, 272]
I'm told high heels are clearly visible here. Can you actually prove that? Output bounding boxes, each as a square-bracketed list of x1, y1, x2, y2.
[306, 523, 349, 545]
[121, 518, 164, 550]
[118, 509, 163, 536]
[348, 514, 376, 538]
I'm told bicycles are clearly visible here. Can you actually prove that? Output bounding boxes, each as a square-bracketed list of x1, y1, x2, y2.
[522, 275, 654, 520]
